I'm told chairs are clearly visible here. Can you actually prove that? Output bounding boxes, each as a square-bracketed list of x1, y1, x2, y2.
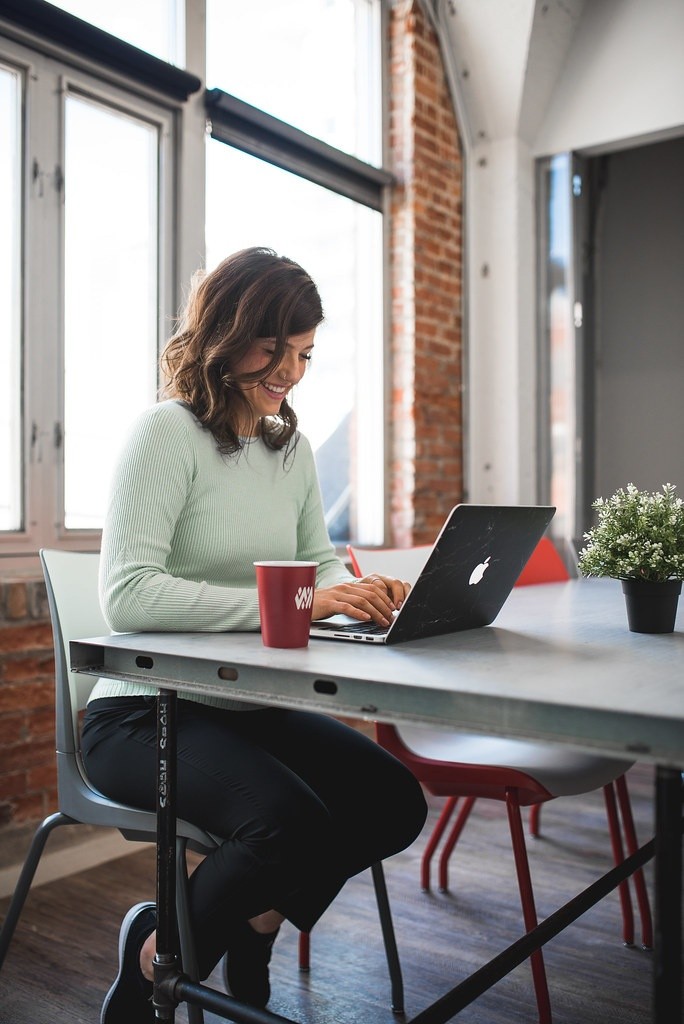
[0, 548, 403, 1024]
[344, 542, 655, 1024]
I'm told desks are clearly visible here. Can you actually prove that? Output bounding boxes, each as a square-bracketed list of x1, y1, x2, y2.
[70, 575, 684, 1024]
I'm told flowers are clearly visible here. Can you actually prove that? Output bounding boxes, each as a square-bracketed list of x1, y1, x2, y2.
[577, 481, 684, 582]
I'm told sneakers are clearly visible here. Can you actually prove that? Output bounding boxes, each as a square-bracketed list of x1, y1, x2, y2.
[99, 902, 162, 1024]
[221, 917, 280, 1008]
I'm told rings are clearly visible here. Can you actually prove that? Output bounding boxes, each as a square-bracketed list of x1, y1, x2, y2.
[369, 578, 381, 585]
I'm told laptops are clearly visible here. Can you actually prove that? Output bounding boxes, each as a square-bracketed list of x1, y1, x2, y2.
[309, 503, 557, 645]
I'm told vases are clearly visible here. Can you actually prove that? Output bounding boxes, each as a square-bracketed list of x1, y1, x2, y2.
[621, 579, 682, 634]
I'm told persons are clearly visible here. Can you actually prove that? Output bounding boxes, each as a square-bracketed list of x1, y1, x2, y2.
[78, 245, 412, 1024]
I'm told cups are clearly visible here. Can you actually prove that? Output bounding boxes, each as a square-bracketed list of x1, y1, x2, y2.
[252, 561, 318, 648]
